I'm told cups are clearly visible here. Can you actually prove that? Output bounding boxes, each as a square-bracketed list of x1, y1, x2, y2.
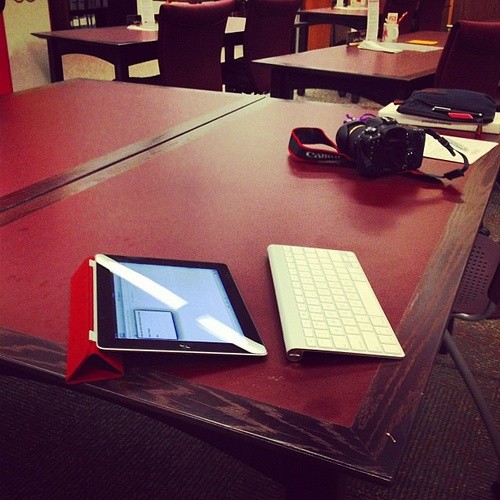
[382, 22, 399, 43]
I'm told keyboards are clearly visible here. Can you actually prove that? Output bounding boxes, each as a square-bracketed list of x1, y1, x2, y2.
[266, 245, 406, 363]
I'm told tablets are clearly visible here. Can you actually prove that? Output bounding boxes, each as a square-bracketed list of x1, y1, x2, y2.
[95, 253, 269, 357]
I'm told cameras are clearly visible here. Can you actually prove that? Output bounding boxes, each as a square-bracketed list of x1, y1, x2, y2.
[337, 116, 429, 179]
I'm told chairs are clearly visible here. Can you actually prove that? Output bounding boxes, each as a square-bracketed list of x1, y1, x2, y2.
[378, 0, 418, 42]
[439, 222, 500, 456]
[128, 0, 234, 91]
[434, 20, 500, 107]
[221, 0, 304, 94]
[418, 0, 448, 34]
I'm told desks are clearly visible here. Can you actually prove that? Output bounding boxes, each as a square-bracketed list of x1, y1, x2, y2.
[0, 79, 268, 207]
[298, 8, 368, 104]
[30, 15, 246, 92]
[0, 96, 499, 499]
[252, 30, 450, 98]
